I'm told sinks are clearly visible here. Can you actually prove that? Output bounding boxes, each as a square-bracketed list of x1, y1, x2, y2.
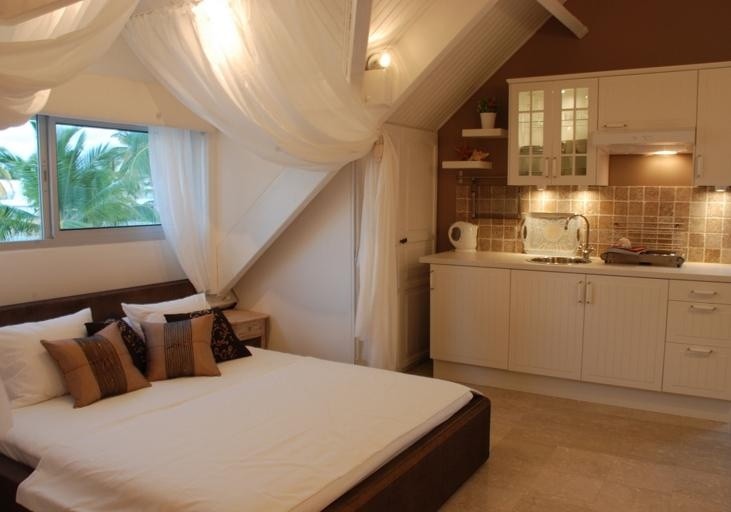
[531, 256, 592, 265]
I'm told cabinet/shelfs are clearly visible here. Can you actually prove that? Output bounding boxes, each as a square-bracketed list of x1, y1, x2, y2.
[694, 61, 731, 186]
[506, 72, 609, 186]
[441, 127, 508, 169]
[387, 123, 439, 371]
[597, 64, 697, 155]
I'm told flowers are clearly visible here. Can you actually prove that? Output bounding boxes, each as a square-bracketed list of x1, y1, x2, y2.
[476, 98, 497, 113]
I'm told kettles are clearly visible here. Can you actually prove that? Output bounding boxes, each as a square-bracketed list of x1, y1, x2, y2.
[447, 220, 480, 252]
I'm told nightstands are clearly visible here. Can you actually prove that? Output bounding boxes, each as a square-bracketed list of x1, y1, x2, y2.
[221, 309, 270, 349]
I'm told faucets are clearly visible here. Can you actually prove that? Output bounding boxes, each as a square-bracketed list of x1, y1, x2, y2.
[564, 214, 593, 261]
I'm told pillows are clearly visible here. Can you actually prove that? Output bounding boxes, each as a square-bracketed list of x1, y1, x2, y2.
[0, 291, 252, 409]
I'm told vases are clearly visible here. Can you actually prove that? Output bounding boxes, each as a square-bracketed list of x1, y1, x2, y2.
[480, 112, 495, 129]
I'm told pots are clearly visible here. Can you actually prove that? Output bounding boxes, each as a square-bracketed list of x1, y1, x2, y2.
[520, 145, 543, 176]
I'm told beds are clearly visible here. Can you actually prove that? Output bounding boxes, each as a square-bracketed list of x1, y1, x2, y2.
[1, 277, 489, 511]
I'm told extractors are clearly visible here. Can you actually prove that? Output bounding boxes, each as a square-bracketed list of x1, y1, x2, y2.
[589, 129, 695, 155]
